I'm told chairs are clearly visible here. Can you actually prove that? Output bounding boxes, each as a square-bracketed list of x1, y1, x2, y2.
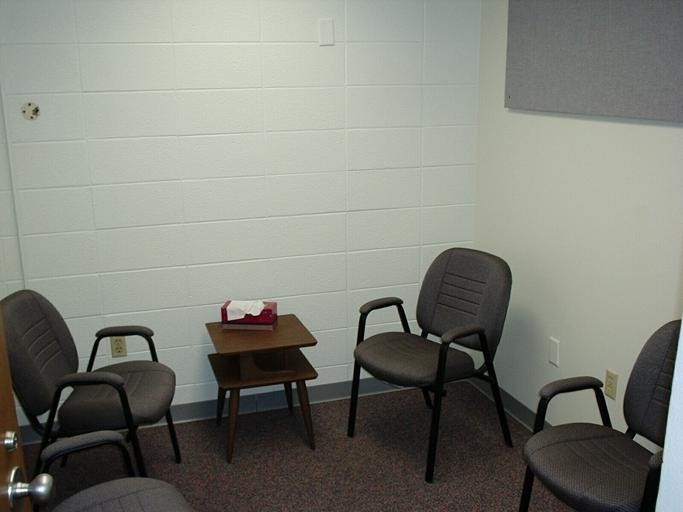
[518, 318, 682, 512]
[31, 429, 195, 512]
[347, 246, 515, 485]
[0, 289, 183, 479]
